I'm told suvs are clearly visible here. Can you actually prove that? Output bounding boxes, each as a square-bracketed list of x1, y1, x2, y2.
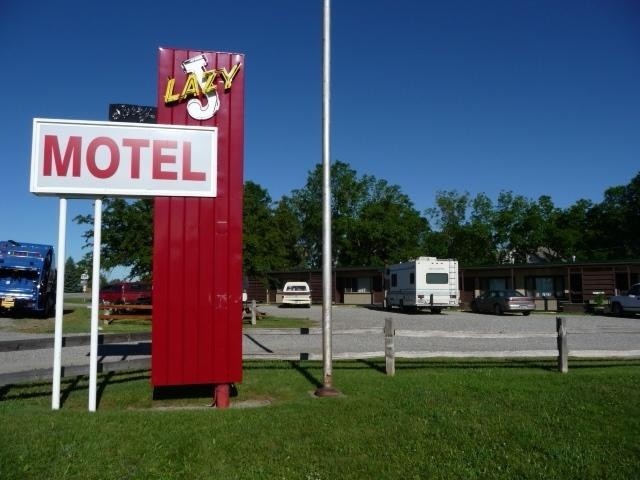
[95, 280, 150, 319]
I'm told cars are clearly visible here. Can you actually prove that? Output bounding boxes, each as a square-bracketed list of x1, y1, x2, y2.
[469, 287, 536, 316]
[610, 281, 640, 317]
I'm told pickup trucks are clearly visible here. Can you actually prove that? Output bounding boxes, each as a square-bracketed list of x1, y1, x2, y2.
[276, 277, 312, 307]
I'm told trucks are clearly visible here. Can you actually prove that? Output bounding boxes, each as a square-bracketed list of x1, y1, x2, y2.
[0, 237, 57, 318]
[382, 255, 463, 314]
